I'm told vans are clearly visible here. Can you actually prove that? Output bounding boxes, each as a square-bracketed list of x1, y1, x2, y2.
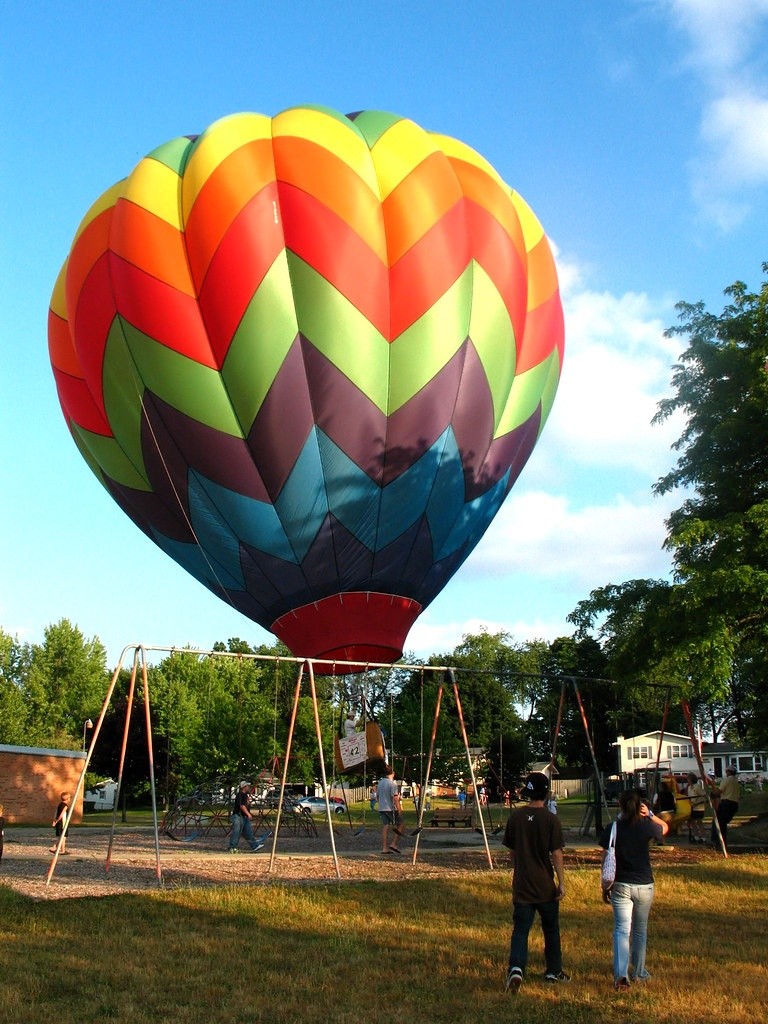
[666, 771, 696, 793]
[632, 770, 646, 793]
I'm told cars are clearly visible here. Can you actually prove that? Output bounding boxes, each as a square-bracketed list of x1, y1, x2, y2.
[290, 796, 348, 817]
[265, 789, 304, 808]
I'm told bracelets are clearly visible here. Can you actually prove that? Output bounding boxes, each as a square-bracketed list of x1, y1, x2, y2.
[648, 810, 654, 819]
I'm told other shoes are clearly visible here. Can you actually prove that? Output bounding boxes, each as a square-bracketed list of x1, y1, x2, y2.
[390, 847, 402, 853]
[381, 852, 393, 854]
[254, 844, 265, 852]
[59, 852, 67, 855]
[49, 850, 55, 853]
[616, 978, 629, 989]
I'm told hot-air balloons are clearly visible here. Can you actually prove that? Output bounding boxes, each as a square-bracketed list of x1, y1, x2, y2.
[47, 100, 564, 780]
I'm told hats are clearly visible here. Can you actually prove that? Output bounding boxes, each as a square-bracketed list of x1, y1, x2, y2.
[724, 765, 737, 772]
[520, 773, 549, 796]
[240, 781, 251, 788]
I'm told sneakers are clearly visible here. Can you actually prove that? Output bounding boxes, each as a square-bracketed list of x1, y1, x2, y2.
[505, 966, 523, 995]
[544, 969, 571, 982]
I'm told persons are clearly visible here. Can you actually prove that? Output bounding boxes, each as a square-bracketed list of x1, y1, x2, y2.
[657, 781, 677, 813]
[49, 792, 71, 855]
[755, 775, 763, 792]
[369, 788, 377, 811]
[377, 767, 405, 855]
[503, 791, 511, 805]
[458, 789, 466, 806]
[228, 780, 265, 852]
[425, 793, 431, 810]
[0, 804, 5, 861]
[344, 712, 364, 737]
[479, 786, 488, 806]
[686, 774, 707, 842]
[547, 795, 559, 816]
[599, 791, 669, 989]
[711, 764, 741, 852]
[501, 773, 572, 991]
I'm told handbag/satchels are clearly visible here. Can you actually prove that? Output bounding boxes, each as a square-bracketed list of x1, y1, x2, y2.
[601, 821, 617, 891]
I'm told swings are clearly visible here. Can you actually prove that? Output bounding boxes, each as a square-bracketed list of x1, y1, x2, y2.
[239, 656, 279, 844]
[521, 676, 551, 811]
[588, 683, 663, 836]
[163, 651, 212, 842]
[391, 668, 424, 838]
[333, 663, 368, 839]
[471, 673, 504, 838]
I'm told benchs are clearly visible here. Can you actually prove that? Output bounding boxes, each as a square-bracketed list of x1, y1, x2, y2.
[430, 807, 473, 828]
